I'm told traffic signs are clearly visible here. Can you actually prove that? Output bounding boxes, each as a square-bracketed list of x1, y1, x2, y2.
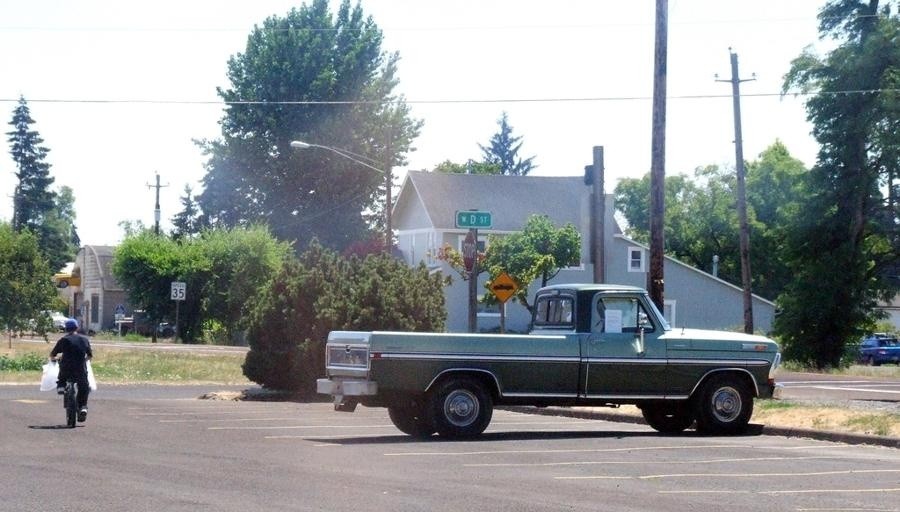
[454, 210, 493, 230]
[170, 282, 187, 301]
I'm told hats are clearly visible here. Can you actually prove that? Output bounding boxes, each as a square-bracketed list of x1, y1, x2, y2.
[65, 321, 76, 329]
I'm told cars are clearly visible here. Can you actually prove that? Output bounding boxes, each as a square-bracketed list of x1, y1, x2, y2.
[29, 309, 78, 332]
[846, 332, 900, 366]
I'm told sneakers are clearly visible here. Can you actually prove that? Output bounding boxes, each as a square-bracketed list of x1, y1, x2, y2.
[78, 405, 87, 421]
[57, 382, 66, 393]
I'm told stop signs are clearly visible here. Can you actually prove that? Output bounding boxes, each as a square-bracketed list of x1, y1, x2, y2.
[462, 232, 476, 272]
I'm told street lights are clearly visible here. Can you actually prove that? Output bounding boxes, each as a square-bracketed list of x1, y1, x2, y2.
[288, 120, 393, 255]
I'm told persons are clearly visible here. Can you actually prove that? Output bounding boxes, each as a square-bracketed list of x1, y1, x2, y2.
[50, 319, 92, 422]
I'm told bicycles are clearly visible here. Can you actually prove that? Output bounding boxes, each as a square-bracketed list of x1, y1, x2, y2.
[50, 353, 91, 429]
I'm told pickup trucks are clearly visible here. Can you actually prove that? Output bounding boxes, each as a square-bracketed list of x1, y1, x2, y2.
[314, 282, 784, 439]
[116, 311, 177, 340]
